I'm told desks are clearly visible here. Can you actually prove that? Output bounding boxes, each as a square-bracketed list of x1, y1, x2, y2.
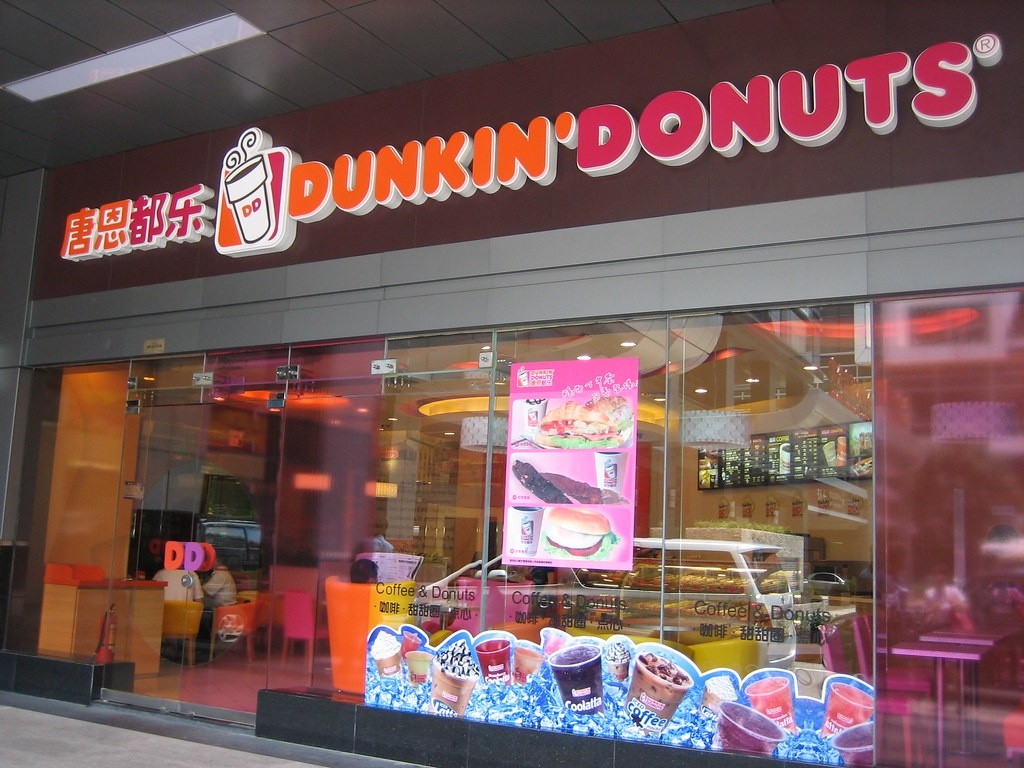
[877, 614, 1024, 768]
[623, 623, 710, 640]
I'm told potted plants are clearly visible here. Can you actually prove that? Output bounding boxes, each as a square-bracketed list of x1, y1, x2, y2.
[414, 552, 448, 583]
[650, 519, 804, 594]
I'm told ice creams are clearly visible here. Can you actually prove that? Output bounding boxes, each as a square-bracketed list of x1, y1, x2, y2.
[603, 642, 631, 681]
[430, 638, 480, 718]
[370, 630, 404, 680]
[697, 675, 737, 725]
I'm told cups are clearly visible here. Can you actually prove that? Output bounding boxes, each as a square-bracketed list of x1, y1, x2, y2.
[700, 681, 738, 722]
[522, 399, 548, 432]
[429, 658, 479, 718]
[403, 630, 423, 657]
[371, 644, 403, 682]
[779, 443, 791, 474]
[542, 628, 566, 654]
[593, 451, 627, 496]
[512, 506, 545, 556]
[405, 651, 434, 686]
[821, 682, 874, 767]
[625, 651, 694, 736]
[607, 662, 629, 681]
[745, 677, 797, 735]
[823, 440, 837, 467]
[715, 700, 785, 757]
[513, 647, 544, 685]
[836, 437, 847, 467]
[475, 640, 512, 686]
[550, 645, 604, 714]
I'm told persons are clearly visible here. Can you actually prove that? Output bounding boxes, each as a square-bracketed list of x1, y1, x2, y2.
[521, 566, 557, 622]
[349, 558, 378, 588]
[917, 525, 1024, 651]
[334, 509, 394, 577]
[141, 539, 240, 667]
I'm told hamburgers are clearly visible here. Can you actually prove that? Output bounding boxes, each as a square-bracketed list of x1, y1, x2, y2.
[541, 506, 617, 559]
[536, 396, 634, 452]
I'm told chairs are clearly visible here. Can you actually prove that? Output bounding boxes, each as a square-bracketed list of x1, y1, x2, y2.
[162, 589, 329, 689]
[564, 619, 759, 680]
[820, 616, 939, 768]
[325, 576, 552, 696]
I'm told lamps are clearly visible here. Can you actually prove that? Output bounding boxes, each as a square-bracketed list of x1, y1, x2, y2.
[680, 344, 751, 452]
[460, 394, 507, 455]
[932, 365, 1019, 445]
